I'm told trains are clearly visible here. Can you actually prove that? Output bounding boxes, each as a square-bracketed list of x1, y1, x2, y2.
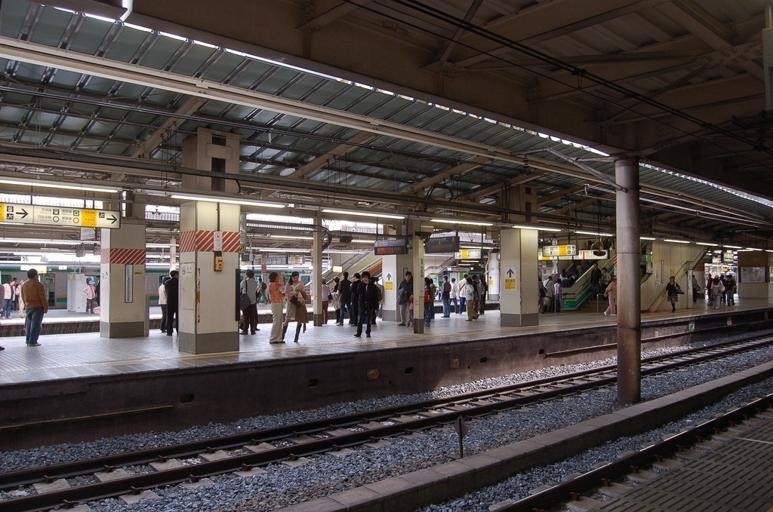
[0, 261, 326, 313]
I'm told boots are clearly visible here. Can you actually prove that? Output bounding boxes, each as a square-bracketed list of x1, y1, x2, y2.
[282, 325, 288, 340]
[294, 323, 302, 342]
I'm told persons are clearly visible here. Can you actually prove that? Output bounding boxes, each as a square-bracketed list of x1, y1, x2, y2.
[241, 269, 307, 343]
[324, 270, 385, 338]
[397, 273, 437, 328]
[434, 273, 486, 322]
[1, 268, 48, 348]
[158, 271, 179, 336]
[665, 275, 681, 313]
[539, 240, 617, 316]
[84, 275, 101, 314]
[692, 272, 737, 309]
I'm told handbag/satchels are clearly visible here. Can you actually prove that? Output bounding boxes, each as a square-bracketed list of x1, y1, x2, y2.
[398, 293, 407, 305]
[240, 294, 249, 309]
[240, 317, 248, 331]
[328, 293, 333, 300]
[540, 285, 546, 297]
[425, 291, 430, 304]
[290, 295, 297, 304]
